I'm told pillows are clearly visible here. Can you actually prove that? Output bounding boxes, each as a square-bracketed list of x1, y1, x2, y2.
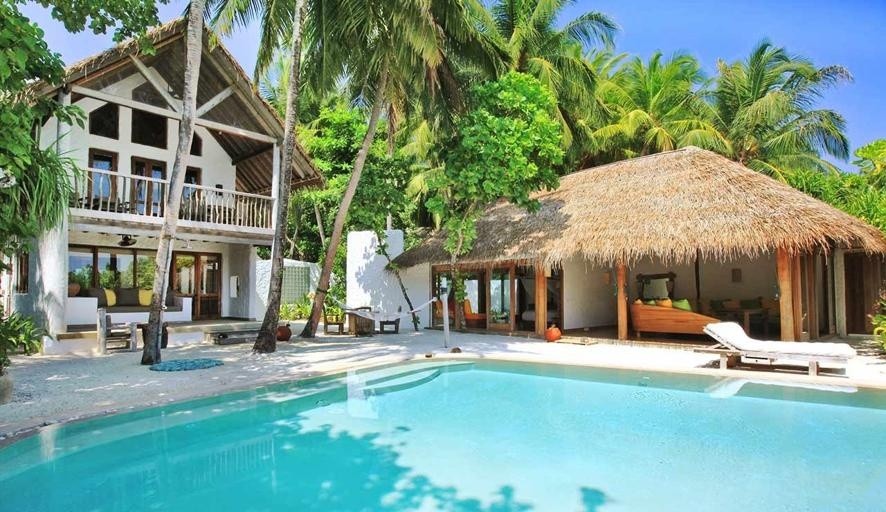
[88, 287, 153, 306]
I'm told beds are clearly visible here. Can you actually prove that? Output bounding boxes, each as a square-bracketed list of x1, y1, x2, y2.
[522, 308, 559, 331]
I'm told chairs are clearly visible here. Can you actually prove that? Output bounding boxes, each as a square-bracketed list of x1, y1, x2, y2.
[67, 190, 247, 226]
[96, 308, 136, 354]
[321, 303, 402, 337]
[693, 321, 859, 379]
[463, 299, 487, 329]
[435, 299, 455, 323]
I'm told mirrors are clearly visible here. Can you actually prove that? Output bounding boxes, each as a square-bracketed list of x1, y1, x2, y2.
[636, 271, 676, 300]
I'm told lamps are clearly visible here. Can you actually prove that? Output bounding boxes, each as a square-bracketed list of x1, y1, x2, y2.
[116, 234, 137, 247]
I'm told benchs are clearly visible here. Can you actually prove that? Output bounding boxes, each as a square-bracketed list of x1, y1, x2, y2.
[97, 296, 192, 324]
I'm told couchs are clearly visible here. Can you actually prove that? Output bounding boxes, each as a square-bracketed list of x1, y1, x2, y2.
[628, 293, 778, 341]
